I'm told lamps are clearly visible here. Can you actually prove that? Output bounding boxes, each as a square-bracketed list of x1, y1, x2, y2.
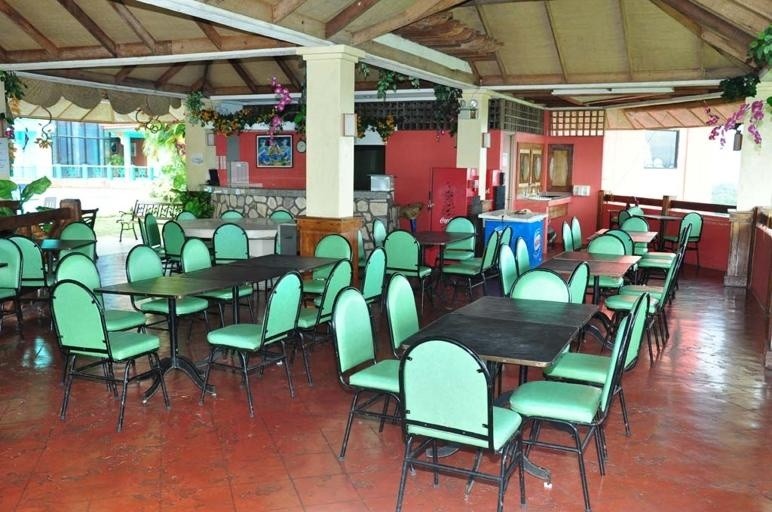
[459, 99, 478, 120]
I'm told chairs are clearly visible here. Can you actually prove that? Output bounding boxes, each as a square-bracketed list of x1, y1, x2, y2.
[600, 253, 682, 368]
[620, 247, 685, 345]
[497, 245, 518, 297]
[462, 227, 512, 292]
[391, 339, 528, 511]
[566, 262, 591, 304]
[434, 216, 477, 284]
[123, 244, 210, 352]
[515, 236, 530, 274]
[505, 314, 632, 512]
[443, 231, 499, 308]
[381, 231, 432, 281]
[303, 233, 352, 293]
[0, 238, 28, 341]
[372, 219, 387, 247]
[329, 286, 413, 461]
[199, 271, 306, 412]
[289, 259, 354, 386]
[8, 235, 58, 316]
[117, 206, 298, 274]
[57, 221, 97, 267]
[536, 292, 652, 464]
[509, 267, 571, 303]
[178, 236, 255, 325]
[52, 252, 157, 388]
[316, 247, 387, 321]
[377, 272, 423, 433]
[357, 230, 369, 267]
[559, 208, 703, 303]
[49, 280, 171, 433]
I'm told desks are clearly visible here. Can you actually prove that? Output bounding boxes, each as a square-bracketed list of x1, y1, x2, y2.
[536, 250, 642, 306]
[91, 253, 348, 403]
[26, 238, 97, 275]
[397, 294, 600, 508]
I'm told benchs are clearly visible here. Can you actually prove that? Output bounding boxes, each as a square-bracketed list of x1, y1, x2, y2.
[117, 199, 183, 222]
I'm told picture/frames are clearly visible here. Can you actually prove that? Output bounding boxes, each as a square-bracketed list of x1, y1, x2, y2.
[513, 141, 574, 196]
[256, 135, 293, 169]
